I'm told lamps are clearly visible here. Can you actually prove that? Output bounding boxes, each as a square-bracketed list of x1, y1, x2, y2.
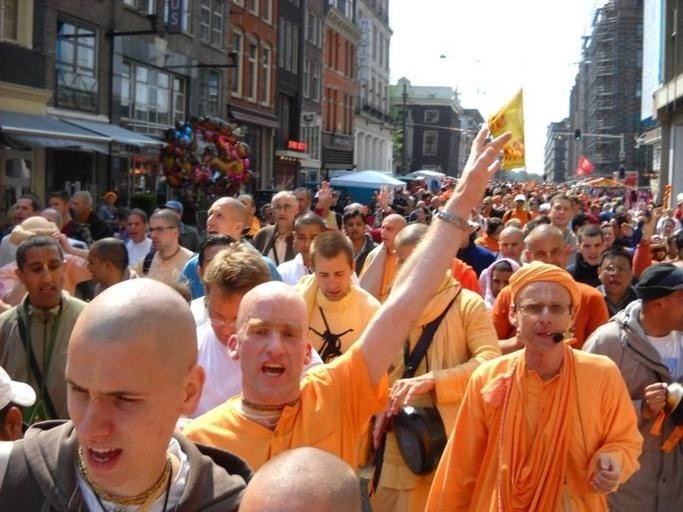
[104, 15, 237, 68]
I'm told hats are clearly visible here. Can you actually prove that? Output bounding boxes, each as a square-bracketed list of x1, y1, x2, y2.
[513, 194, 526, 203]
[159, 200, 183, 211]
[633, 262, 683, 300]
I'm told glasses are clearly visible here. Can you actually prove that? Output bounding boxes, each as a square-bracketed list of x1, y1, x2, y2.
[514, 301, 572, 316]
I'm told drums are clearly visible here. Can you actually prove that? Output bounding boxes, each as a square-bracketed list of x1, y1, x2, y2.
[393, 401, 448, 474]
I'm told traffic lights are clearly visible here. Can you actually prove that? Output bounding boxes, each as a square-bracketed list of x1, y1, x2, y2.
[619, 167, 624, 179]
[575, 129, 580, 142]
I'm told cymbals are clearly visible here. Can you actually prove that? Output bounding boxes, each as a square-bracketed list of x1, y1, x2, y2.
[660, 382, 683, 418]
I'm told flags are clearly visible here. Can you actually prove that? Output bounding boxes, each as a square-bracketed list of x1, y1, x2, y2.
[488, 88, 525, 170]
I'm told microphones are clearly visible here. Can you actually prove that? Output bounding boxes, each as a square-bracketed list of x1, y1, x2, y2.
[553, 314, 573, 343]
